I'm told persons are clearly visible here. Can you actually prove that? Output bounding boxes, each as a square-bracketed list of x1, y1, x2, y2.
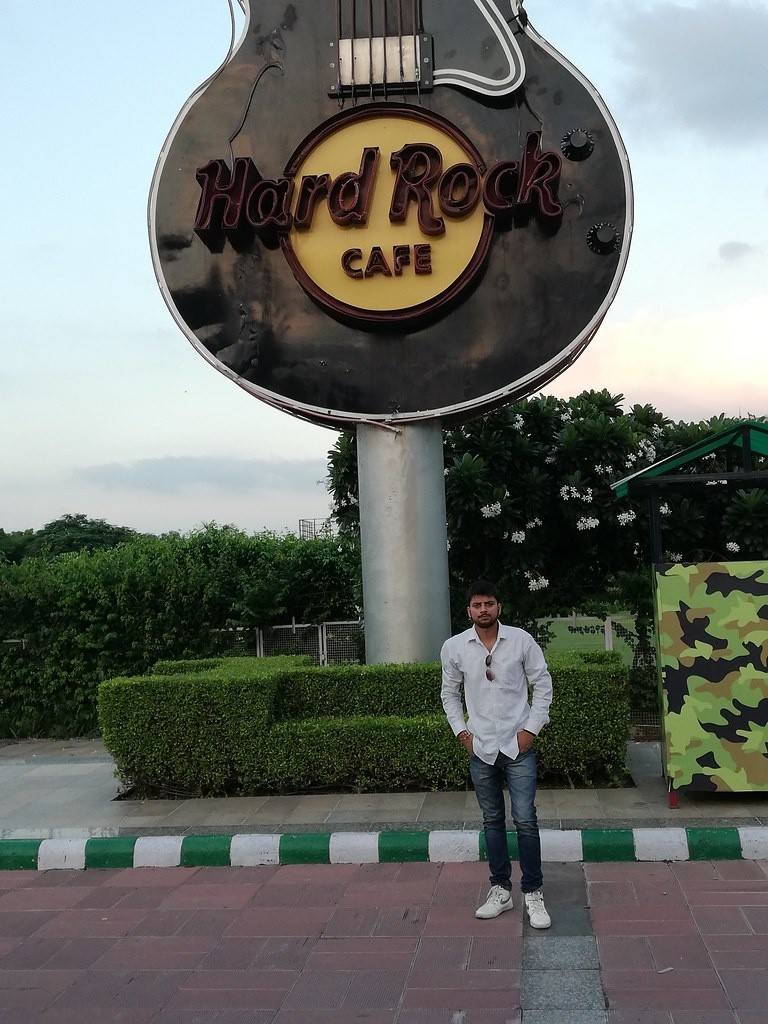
[440, 580, 553, 929]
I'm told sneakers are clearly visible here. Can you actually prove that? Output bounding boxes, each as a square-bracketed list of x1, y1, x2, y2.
[525, 881, 552, 928]
[475, 885, 518, 918]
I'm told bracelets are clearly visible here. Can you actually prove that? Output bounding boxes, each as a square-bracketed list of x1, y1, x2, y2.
[459, 730, 471, 741]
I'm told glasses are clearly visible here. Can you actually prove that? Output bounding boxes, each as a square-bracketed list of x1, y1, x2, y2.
[486, 654, 495, 682]
